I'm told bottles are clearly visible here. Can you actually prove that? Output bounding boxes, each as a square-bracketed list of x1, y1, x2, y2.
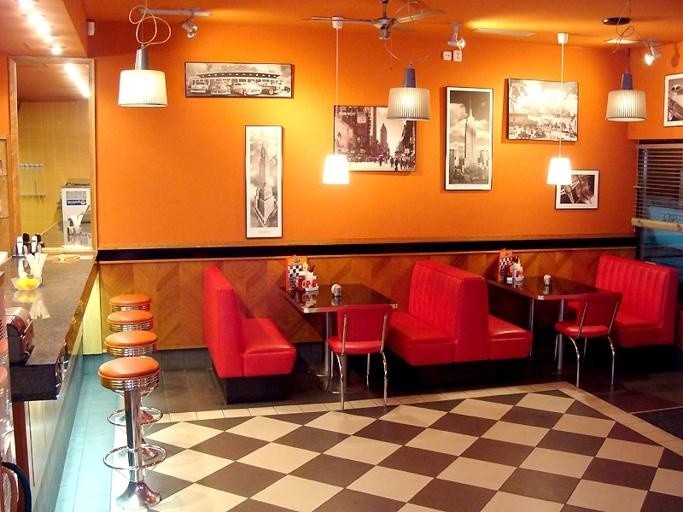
[543, 274, 552, 285]
[313, 276, 316, 290]
[14, 232, 45, 254]
[304, 272, 312, 290]
[297, 276, 305, 291]
[512, 264, 523, 278]
[334, 285, 341, 296]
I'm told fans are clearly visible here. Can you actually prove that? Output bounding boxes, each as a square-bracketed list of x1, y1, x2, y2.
[305, 0, 447, 39]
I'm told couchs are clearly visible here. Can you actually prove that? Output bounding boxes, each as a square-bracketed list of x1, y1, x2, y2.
[388, 262, 531, 384]
[595, 255, 676, 348]
[201, 265, 297, 404]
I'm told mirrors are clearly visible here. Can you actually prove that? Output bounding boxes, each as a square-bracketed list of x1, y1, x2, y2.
[7, 55, 99, 257]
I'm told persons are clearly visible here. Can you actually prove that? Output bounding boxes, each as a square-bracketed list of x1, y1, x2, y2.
[375, 155, 414, 170]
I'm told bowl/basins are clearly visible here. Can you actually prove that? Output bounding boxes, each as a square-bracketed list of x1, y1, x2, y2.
[11, 274, 43, 290]
[11, 291, 40, 304]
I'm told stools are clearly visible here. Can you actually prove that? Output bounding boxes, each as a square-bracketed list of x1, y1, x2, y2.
[98, 293, 161, 510]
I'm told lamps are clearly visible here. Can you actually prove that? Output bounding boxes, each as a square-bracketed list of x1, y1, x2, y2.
[448, 24, 464, 48]
[645, 40, 661, 64]
[548, 31, 572, 185]
[607, 1, 646, 122]
[387, 61, 430, 120]
[118, 1, 167, 108]
[323, 15, 349, 185]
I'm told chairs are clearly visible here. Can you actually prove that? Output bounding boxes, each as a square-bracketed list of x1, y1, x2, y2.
[556, 293, 622, 390]
[663, 74, 683, 127]
[324, 304, 392, 410]
[245, 125, 282, 238]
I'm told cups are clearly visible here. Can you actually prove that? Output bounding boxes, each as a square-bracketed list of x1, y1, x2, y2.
[506, 278, 511, 285]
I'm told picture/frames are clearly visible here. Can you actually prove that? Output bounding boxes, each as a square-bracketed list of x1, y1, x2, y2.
[186, 60, 294, 99]
[555, 169, 599, 210]
[445, 86, 494, 191]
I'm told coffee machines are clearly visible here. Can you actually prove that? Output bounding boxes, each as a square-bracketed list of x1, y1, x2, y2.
[60, 183, 92, 251]
